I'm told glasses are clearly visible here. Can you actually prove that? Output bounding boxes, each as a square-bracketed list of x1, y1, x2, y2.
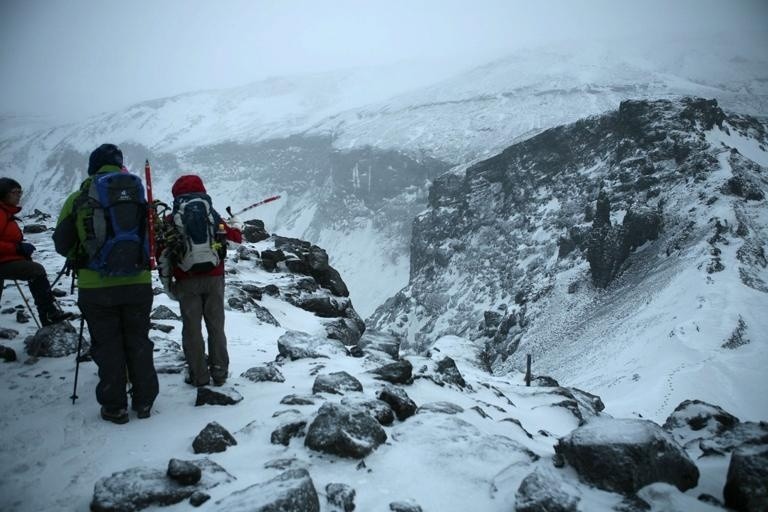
[8, 188, 24, 198]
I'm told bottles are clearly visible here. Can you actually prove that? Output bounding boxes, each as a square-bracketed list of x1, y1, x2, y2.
[217, 223, 226, 233]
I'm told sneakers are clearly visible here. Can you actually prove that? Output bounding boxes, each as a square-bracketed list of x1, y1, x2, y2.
[184, 376, 210, 387]
[214, 376, 226, 388]
[131, 403, 151, 419]
[36, 306, 74, 327]
[100, 405, 131, 424]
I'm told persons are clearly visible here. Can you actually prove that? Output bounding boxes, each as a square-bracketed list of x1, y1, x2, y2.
[0, 177, 73, 326]
[52, 144, 158, 425]
[156, 174, 242, 388]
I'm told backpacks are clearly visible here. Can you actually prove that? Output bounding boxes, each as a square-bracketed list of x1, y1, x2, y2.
[82, 170, 153, 279]
[170, 191, 227, 275]
